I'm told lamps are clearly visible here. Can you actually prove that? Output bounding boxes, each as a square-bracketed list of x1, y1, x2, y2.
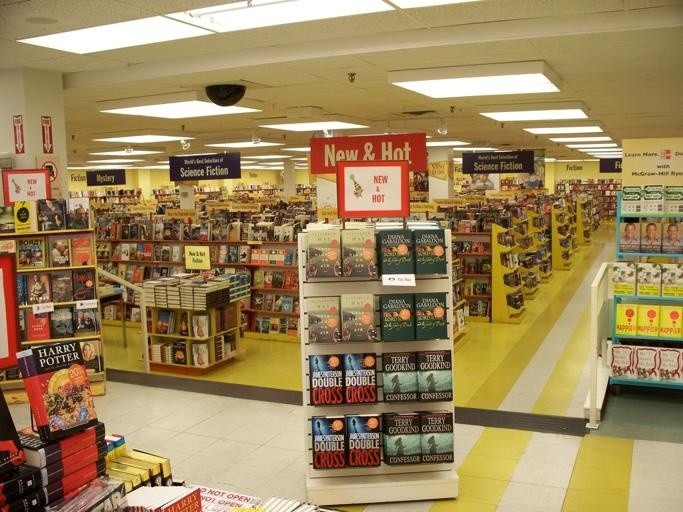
[437, 122, 448, 136]
[251, 134, 261, 144]
[180, 140, 191, 151]
[125, 145, 134, 154]
[324, 129, 332, 137]
[384, 127, 395, 135]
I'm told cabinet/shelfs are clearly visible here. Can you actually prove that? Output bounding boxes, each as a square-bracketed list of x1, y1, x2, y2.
[582, 190, 682, 429]
[139, 295, 249, 377]
[70, 181, 621, 344]
[1, 211, 108, 404]
[293, 225, 461, 507]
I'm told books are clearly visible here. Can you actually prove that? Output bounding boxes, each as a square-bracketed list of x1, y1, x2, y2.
[1, 196, 102, 374]
[142, 270, 250, 366]
[602, 184, 682, 382]
[94, 198, 317, 335]
[1, 339, 346, 511]
[444, 185, 602, 318]
[305, 222, 454, 472]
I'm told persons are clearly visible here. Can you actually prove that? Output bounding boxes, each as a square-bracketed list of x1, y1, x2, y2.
[471, 173, 494, 189]
